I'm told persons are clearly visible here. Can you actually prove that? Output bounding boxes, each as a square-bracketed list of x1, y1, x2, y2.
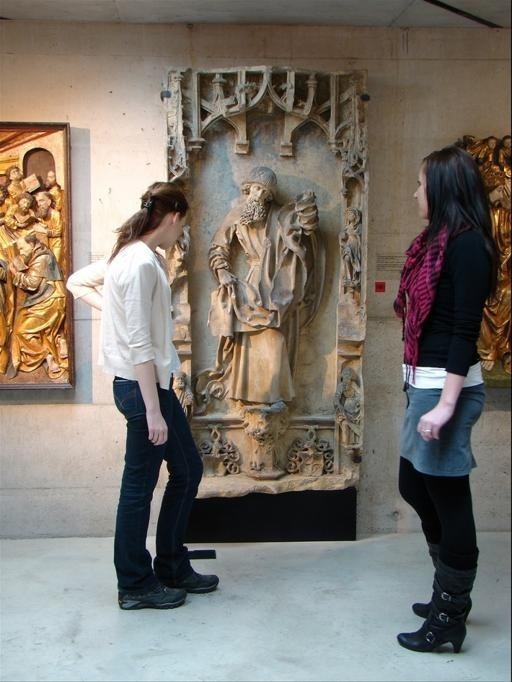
[63, 181, 221, 612]
[193, 166, 324, 415]
[391, 145, 503, 655]
[0, 166, 69, 378]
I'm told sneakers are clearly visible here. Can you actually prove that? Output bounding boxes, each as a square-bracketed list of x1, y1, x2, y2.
[155, 563, 221, 595]
[117, 580, 188, 611]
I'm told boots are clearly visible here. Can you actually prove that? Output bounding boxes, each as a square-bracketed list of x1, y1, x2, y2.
[394, 575, 470, 655]
[410, 569, 472, 625]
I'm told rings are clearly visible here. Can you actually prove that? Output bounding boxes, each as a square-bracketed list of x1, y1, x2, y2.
[425, 429, 431, 433]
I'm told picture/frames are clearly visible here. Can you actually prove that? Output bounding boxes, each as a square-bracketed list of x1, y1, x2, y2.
[0, 121, 76, 391]
